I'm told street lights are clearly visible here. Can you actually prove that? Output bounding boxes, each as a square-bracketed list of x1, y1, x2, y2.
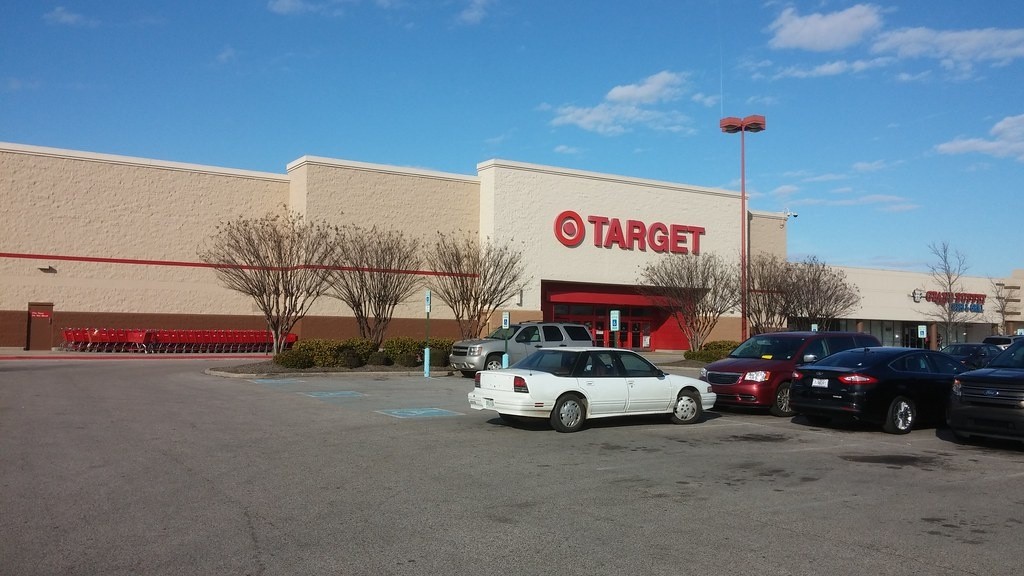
[720, 110, 766, 342]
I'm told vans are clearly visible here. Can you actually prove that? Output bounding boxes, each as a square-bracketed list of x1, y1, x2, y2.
[450, 320, 595, 377]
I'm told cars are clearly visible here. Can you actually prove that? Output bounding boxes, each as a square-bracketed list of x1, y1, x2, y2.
[469, 346, 717, 437]
[701, 328, 883, 418]
[789, 343, 968, 434]
[931, 325, 1024, 452]
